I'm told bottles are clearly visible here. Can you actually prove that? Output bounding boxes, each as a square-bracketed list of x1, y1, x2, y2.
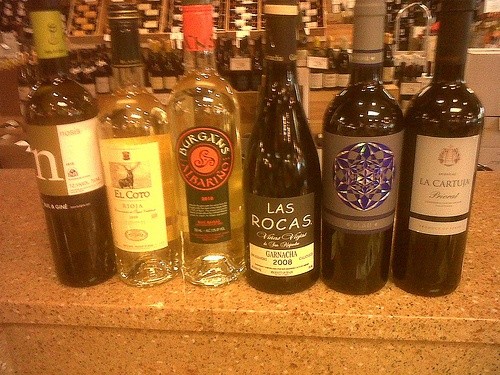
[321, 0, 405, 296]
[242, 0, 321, 296]
[165, 0, 248, 286]
[22, 0, 117, 287]
[392, 0, 485, 297]
[96, 0, 183, 288]
[1, 0, 434, 114]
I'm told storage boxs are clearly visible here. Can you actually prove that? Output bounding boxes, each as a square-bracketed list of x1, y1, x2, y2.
[66, 0, 327, 44]
